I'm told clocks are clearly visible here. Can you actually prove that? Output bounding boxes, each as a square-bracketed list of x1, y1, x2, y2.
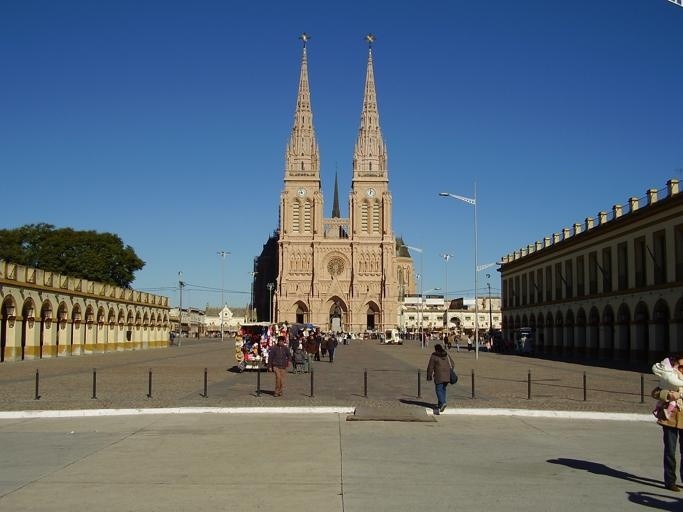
[365, 187, 376, 198]
[296, 187, 306, 199]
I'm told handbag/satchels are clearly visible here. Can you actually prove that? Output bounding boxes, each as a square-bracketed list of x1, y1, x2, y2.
[450, 368, 458, 384]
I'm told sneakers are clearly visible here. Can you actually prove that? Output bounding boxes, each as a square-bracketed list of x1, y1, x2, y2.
[666, 485, 680, 492]
[664, 409, 670, 420]
[440, 404, 447, 412]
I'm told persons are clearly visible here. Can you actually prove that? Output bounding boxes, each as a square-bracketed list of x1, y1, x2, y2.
[651, 351, 682, 491]
[424, 332, 430, 347]
[268, 336, 292, 398]
[426, 344, 455, 412]
[285, 328, 351, 375]
[453, 334, 460, 352]
[443, 335, 448, 350]
[652, 356, 682, 421]
[466, 334, 472, 353]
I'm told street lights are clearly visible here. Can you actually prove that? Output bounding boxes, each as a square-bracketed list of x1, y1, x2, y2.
[216, 252, 230, 341]
[248, 272, 259, 320]
[401, 245, 423, 337]
[437, 193, 481, 360]
[440, 254, 453, 341]
[266, 282, 276, 322]
